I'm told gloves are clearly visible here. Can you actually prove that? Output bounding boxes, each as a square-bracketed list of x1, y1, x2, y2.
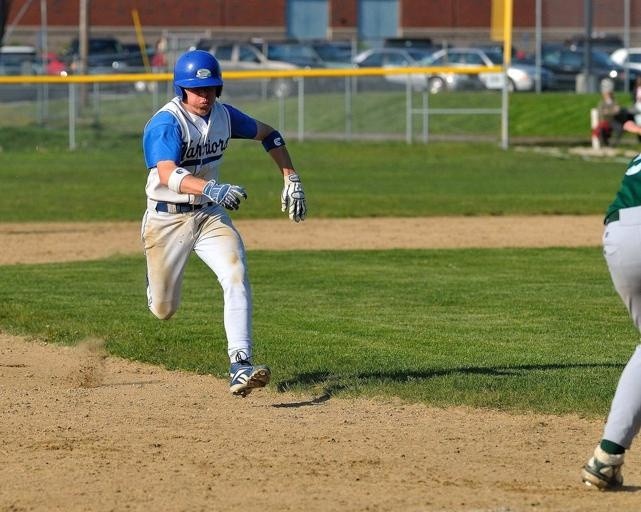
[201, 179, 248, 210]
[280, 172, 309, 223]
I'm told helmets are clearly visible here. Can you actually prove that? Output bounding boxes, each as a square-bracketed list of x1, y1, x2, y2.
[173, 49, 225, 104]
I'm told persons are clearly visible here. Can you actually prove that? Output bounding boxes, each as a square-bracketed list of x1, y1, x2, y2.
[43, 53, 65, 75]
[138, 50, 306, 396]
[600, 74, 641, 137]
[152, 37, 172, 67]
[579, 152, 641, 494]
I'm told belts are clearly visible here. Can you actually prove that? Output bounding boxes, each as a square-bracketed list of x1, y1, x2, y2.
[155, 200, 215, 214]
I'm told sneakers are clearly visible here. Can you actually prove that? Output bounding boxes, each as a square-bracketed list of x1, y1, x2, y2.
[229, 360, 273, 398]
[580, 444, 628, 493]
